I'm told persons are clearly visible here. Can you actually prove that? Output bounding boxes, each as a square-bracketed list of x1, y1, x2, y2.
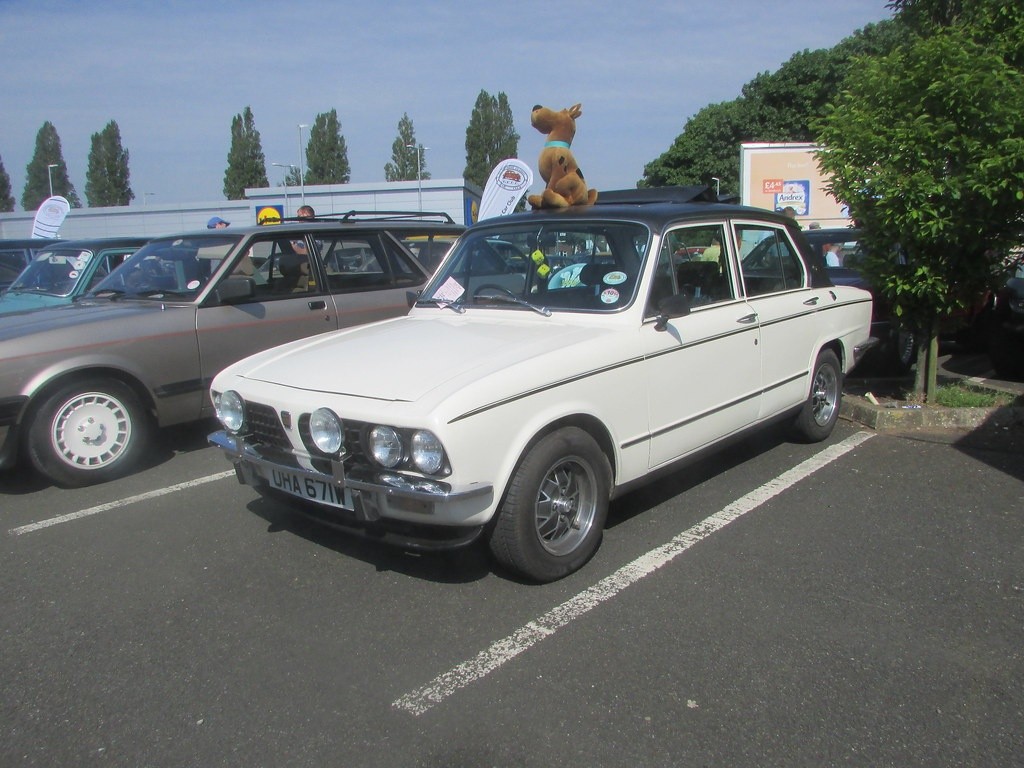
[701, 234, 723, 275]
[289, 205, 325, 257]
[808, 222, 846, 267]
[205, 216, 232, 273]
[755, 206, 799, 269]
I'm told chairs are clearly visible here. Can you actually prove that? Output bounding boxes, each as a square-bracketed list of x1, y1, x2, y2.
[270, 254, 313, 295]
[216, 257, 257, 298]
[842, 253, 858, 266]
[580, 264, 620, 309]
[665, 262, 720, 311]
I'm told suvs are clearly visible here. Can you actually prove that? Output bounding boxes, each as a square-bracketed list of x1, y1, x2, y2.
[1, 210, 535, 491]
[353, 237, 545, 301]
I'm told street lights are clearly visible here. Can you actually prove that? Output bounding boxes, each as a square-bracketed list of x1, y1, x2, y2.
[271, 162, 295, 219]
[406, 144, 431, 212]
[297, 124, 310, 206]
[142, 192, 155, 207]
[711, 177, 720, 196]
[47, 164, 60, 197]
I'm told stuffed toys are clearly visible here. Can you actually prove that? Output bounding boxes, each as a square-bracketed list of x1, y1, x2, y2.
[527, 103, 598, 207]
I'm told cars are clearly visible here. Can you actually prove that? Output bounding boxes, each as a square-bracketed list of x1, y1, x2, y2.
[740, 227, 923, 377]
[502, 245, 726, 285]
[0, 238, 70, 297]
[203, 201, 884, 585]
[0, 235, 204, 319]
[251, 251, 285, 284]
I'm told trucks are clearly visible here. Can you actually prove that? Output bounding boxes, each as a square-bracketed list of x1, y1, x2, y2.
[737, 139, 887, 231]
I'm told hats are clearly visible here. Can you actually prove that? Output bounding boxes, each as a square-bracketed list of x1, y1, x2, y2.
[207, 217, 230, 229]
